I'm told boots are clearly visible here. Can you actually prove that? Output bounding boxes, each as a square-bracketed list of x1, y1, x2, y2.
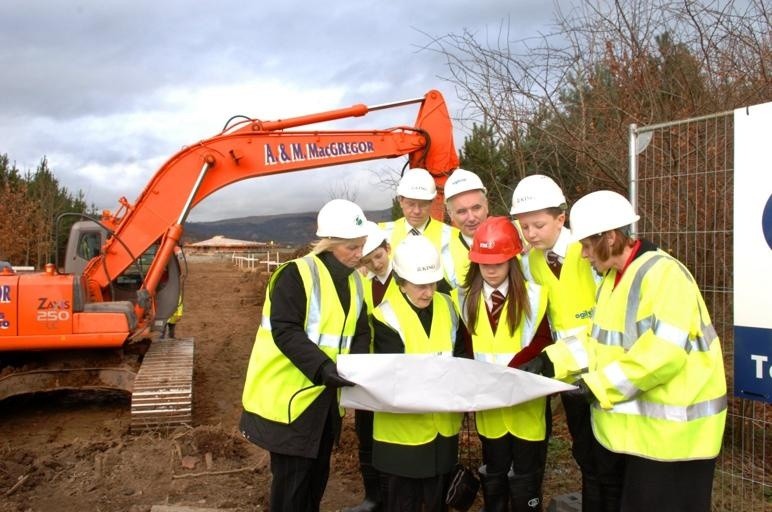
[338, 464, 386, 511]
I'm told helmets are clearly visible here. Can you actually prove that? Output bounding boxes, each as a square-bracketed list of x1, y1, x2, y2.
[569, 190, 641, 244]
[392, 236, 444, 286]
[468, 216, 523, 265]
[316, 198, 368, 240]
[509, 173, 567, 217]
[395, 169, 438, 201]
[444, 169, 488, 202]
[360, 221, 389, 257]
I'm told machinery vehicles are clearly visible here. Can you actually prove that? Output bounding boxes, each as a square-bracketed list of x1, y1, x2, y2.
[1, 81, 461, 428]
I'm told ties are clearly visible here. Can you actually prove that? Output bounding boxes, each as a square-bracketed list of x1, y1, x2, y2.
[490, 290, 505, 330]
[546, 251, 561, 279]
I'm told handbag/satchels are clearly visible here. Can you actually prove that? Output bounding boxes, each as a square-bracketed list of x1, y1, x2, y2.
[443, 463, 480, 512]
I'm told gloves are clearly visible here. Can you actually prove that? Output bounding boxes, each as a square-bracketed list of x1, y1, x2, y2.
[566, 378, 594, 405]
[518, 352, 553, 378]
[318, 362, 356, 388]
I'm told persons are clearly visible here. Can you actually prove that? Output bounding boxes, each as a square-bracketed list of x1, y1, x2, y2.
[366, 236, 473, 510]
[355, 220, 395, 511]
[515, 189, 728, 512]
[442, 168, 534, 295]
[159, 295, 183, 338]
[359, 168, 462, 277]
[448, 215, 555, 511]
[239, 198, 371, 512]
[510, 173, 611, 512]
